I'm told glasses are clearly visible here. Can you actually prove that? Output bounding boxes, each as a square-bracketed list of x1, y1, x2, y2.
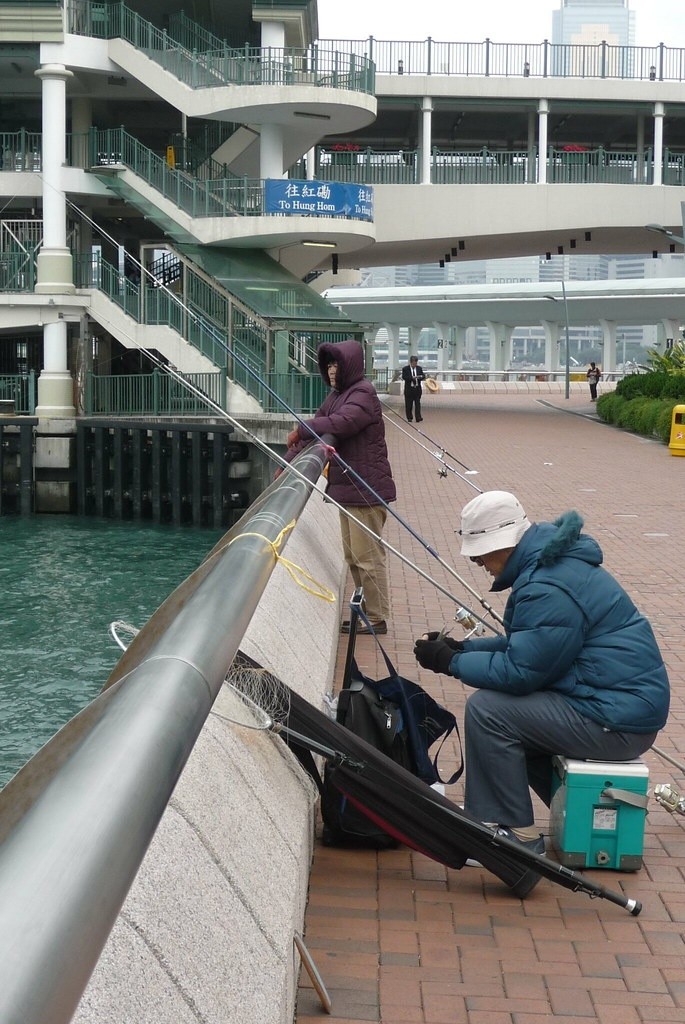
[469, 556, 485, 567]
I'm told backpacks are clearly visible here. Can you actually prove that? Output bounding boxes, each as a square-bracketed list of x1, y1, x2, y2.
[320, 681, 411, 851]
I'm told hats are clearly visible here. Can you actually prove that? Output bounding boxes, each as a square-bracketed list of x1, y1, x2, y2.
[459, 490, 532, 557]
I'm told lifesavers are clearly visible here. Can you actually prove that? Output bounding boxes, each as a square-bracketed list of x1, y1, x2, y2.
[426, 378, 440, 392]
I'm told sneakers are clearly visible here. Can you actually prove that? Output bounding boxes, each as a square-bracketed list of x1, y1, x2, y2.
[465, 822, 546, 867]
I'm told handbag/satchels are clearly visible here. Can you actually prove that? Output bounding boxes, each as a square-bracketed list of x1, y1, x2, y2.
[344, 602, 464, 786]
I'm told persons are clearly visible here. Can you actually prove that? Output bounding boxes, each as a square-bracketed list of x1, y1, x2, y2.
[402, 356, 425, 422]
[413, 490, 671, 867]
[272, 339, 397, 634]
[587, 362, 601, 402]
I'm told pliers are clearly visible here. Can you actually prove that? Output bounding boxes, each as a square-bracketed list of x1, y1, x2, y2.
[437, 624, 454, 641]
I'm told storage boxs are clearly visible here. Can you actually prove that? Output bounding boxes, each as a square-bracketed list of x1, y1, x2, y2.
[549, 755, 649, 869]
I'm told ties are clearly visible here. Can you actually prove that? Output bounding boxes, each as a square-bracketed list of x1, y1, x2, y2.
[412, 367, 416, 388]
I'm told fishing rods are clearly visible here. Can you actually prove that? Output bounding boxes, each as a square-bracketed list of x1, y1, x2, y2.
[23, 154, 685, 777]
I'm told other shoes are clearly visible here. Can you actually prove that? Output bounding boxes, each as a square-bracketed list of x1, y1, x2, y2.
[416, 418, 423, 422]
[343, 621, 350, 626]
[341, 620, 387, 634]
[408, 418, 412, 422]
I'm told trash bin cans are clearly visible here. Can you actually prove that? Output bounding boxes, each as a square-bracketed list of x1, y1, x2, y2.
[398, 60, 403, 75]
[668, 405, 685, 457]
[524, 62, 530, 77]
[650, 66, 656, 81]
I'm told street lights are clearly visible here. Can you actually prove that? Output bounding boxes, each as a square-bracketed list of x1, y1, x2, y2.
[543, 294, 569, 399]
